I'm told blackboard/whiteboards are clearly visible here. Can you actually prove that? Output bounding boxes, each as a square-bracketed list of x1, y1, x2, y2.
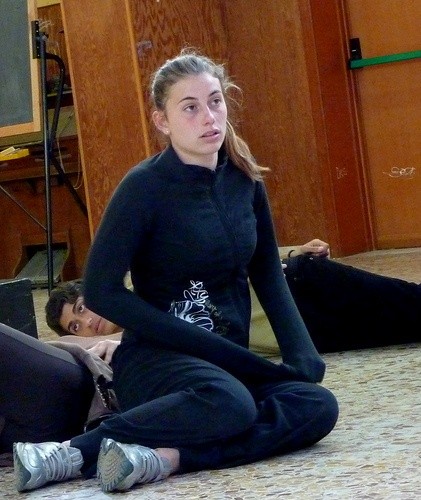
[0, 0, 46, 151]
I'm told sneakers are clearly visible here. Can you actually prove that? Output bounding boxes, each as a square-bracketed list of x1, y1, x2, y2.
[96, 438, 164, 493]
[11, 439, 84, 493]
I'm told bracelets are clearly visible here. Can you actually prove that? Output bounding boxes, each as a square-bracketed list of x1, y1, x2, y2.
[285, 248, 296, 259]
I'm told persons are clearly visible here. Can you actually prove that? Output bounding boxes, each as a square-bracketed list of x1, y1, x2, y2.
[29, 237, 421, 354]
[7, 53, 336, 493]
